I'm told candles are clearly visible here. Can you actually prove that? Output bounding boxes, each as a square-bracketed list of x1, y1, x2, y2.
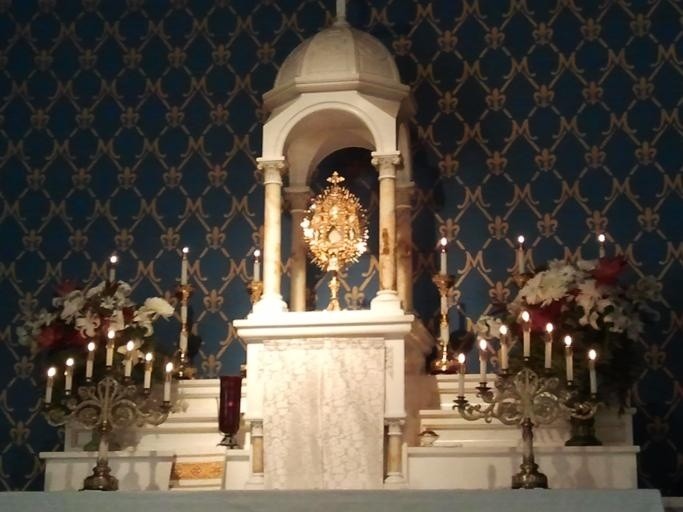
[253, 259, 260, 281]
[441, 246, 446, 275]
[45, 328, 172, 408]
[457, 310, 596, 396]
[518, 243, 524, 274]
[109, 268, 116, 283]
[600, 242, 604, 257]
[181, 258, 187, 285]
